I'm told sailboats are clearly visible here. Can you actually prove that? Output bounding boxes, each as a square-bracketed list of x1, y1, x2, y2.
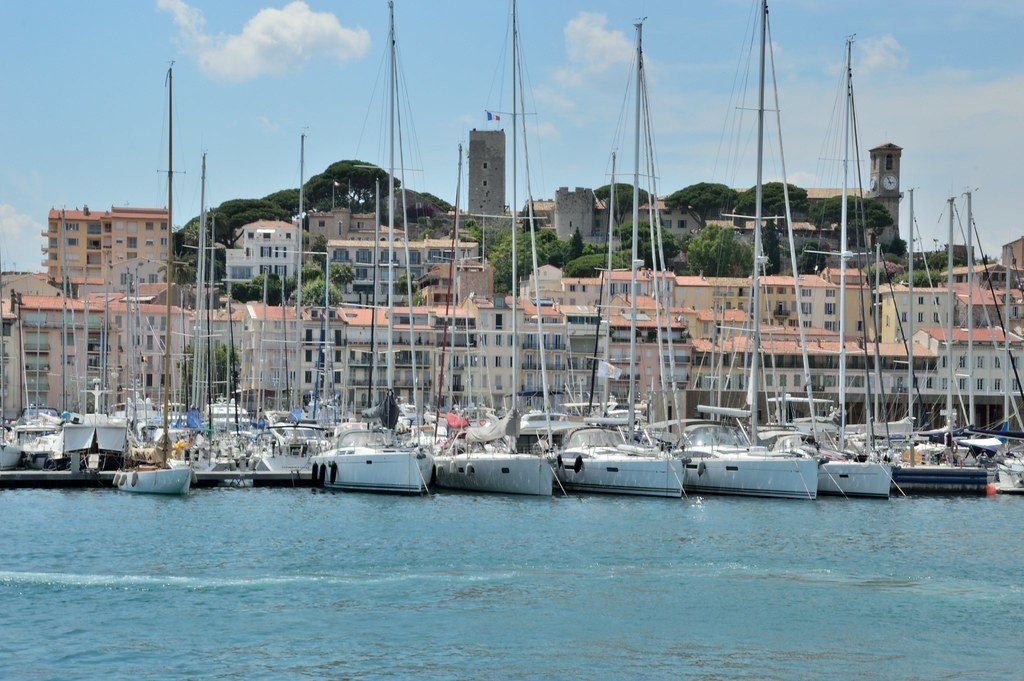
[0, 0, 1023, 500]
